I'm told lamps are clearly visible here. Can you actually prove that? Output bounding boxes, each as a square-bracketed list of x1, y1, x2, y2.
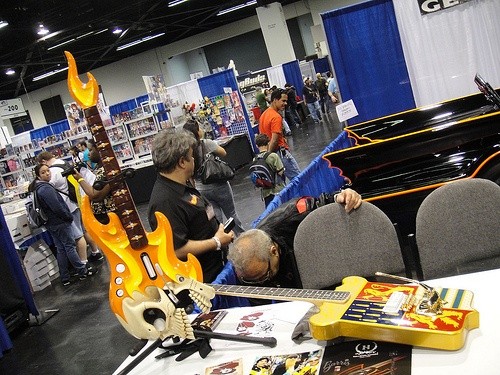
[32, 65, 68, 82]
[168, 0, 189, 8]
[216, 0, 258, 17]
[111, 27, 168, 53]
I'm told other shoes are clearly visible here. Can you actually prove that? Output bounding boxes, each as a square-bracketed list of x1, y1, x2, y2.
[86, 262, 96, 270]
[88, 252, 104, 262]
[79, 268, 99, 280]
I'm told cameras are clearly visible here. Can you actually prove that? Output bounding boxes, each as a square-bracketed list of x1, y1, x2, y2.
[61, 162, 87, 177]
[223, 216, 235, 234]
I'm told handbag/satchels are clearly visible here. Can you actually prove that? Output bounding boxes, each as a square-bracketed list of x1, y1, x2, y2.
[196, 140, 236, 185]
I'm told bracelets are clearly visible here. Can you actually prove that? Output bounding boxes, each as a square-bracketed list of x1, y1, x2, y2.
[212, 237, 221, 250]
[330, 95, 333, 97]
[77, 177, 85, 183]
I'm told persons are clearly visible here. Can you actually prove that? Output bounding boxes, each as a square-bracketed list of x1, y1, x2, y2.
[252, 134, 286, 209]
[29, 138, 113, 286]
[255, 72, 342, 136]
[228, 188, 362, 307]
[147, 119, 246, 284]
[258, 88, 301, 186]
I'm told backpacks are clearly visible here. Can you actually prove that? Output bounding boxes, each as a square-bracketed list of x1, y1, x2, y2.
[248, 152, 277, 189]
[24, 191, 49, 230]
[49, 159, 77, 203]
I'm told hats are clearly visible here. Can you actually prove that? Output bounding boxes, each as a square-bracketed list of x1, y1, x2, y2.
[303, 77, 309, 83]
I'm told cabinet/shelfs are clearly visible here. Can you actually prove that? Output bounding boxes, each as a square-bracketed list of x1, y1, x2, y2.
[0, 70, 269, 294]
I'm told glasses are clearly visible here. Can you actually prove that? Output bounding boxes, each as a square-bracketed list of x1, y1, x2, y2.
[240, 261, 273, 286]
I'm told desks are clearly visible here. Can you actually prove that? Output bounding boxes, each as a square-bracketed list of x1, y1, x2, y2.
[112, 268, 500, 375]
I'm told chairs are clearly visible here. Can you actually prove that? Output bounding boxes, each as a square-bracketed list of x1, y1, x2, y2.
[414, 178, 500, 279]
[293, 202, 406, 289]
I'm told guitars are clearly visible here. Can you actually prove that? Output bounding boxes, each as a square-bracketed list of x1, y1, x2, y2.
[66, 49, 204, 341]
[172, 274, 479, 351]
